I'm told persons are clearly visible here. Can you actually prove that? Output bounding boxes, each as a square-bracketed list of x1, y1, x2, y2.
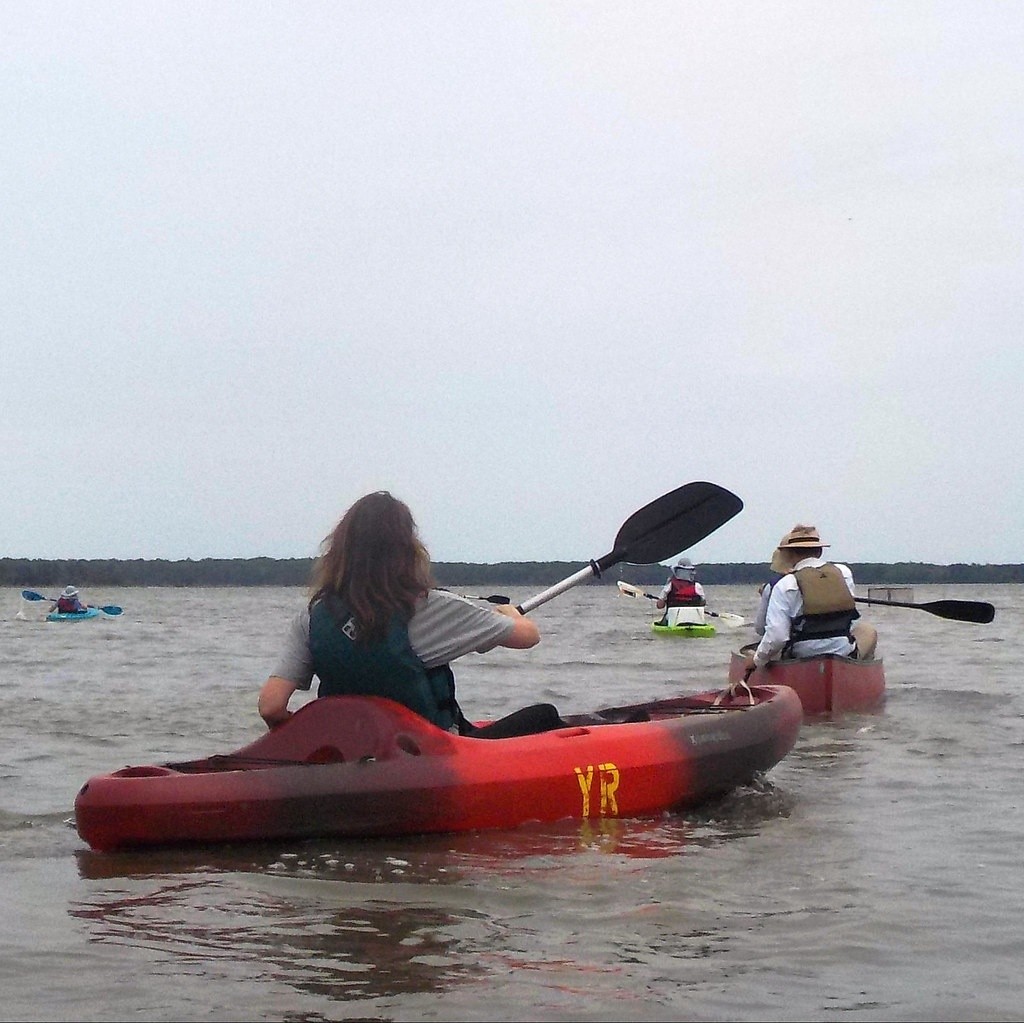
[746, 523, 878, 671]
[657, 558, 706, 618]
[48, 586, 87, 614]
[258, 491, 652, 740]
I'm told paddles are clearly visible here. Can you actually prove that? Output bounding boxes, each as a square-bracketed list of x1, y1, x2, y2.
[614, 576, 747, 628]
[852, 594, 996, 626]
[515, 477, 744, 621]
[461, 593, 511, 606]
[19, 589, 123, 617]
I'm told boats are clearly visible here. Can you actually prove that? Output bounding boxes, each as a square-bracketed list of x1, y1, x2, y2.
[728, 639, 887, 719]
[651, 619, 716, 639]
[74, 684, 803, 854]
[46, 606, 100, 622]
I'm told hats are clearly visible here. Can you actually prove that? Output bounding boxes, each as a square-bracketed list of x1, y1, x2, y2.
[778, 522, 830, 551]
[60, 585, 79, 599]
[670, 557, 696, 581]
[770, 528, 793, 574]
